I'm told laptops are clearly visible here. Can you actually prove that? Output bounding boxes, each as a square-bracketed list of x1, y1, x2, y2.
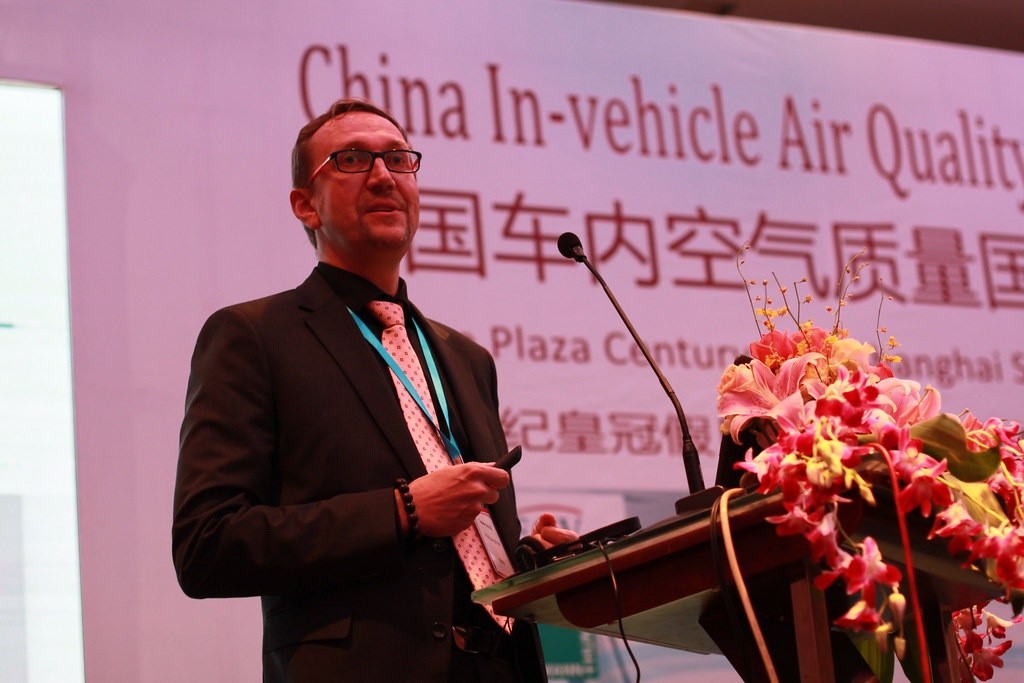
[714, 356, 775, 494]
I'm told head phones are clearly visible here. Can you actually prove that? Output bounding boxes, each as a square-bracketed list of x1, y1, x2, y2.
[511, 516, 641, 575]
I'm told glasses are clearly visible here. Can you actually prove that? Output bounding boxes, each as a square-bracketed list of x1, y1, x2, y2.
[304, 150, 422, 187]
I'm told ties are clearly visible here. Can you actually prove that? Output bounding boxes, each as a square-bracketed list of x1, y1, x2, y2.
[368, 301, 516, 635]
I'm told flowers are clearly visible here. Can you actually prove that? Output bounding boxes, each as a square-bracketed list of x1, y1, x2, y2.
[718, 239, 1024, 683]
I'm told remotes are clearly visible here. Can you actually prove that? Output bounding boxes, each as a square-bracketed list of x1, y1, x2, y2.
[491, 446, 524, 471]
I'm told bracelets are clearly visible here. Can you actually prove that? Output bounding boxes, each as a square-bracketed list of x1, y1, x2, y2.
[394, 478, 421, 540]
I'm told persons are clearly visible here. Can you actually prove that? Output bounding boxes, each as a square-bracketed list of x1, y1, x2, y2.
[171, 100, 580, 683]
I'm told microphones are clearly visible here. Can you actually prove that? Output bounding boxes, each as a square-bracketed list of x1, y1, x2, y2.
[558, 232, 728, 516]
